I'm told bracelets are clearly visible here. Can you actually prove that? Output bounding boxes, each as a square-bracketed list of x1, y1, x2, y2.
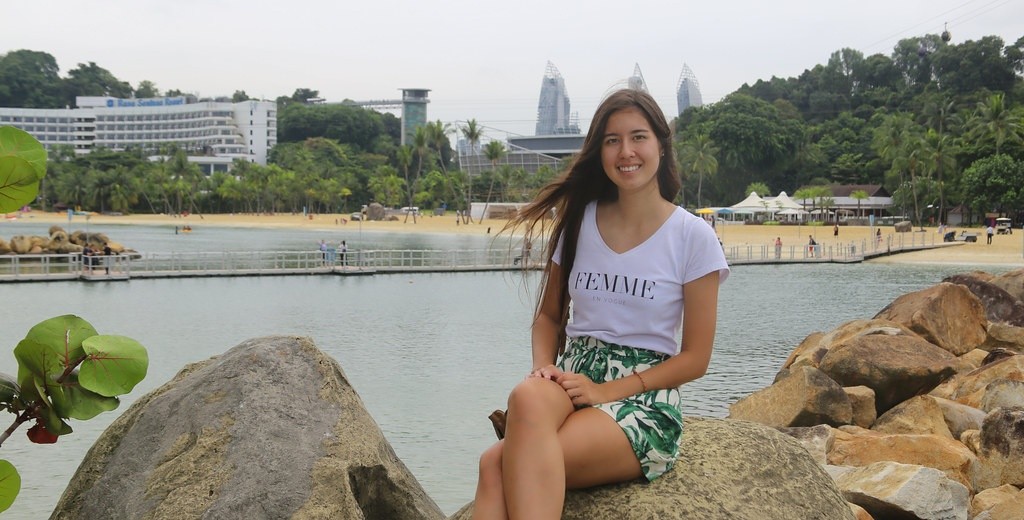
[634, 371, 647, 393]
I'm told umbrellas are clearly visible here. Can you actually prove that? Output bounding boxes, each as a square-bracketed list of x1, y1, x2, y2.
[695, 191, 835, 224]
[947, 202, 982, 223]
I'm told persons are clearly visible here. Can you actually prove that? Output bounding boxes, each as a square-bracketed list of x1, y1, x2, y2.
[319, 238, 328, 265]
[456, 210, 460, 225]
[709, 215, 716, 228]
[877, 228, 882, 249]
[775, 237, 782, 259]
[833, 224, 839, 238]
[944, 230, 956, 242]
[471, 89, 730, 520]
[808, 235, 814, 258]
[338, 240, 348, 266]
[83, 242, 111, 275]
[985, 221, 1012, 245]
[521, 237, 533, 267]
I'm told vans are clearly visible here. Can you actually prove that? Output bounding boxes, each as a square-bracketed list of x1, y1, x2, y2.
[400, 206, 419, 215]
